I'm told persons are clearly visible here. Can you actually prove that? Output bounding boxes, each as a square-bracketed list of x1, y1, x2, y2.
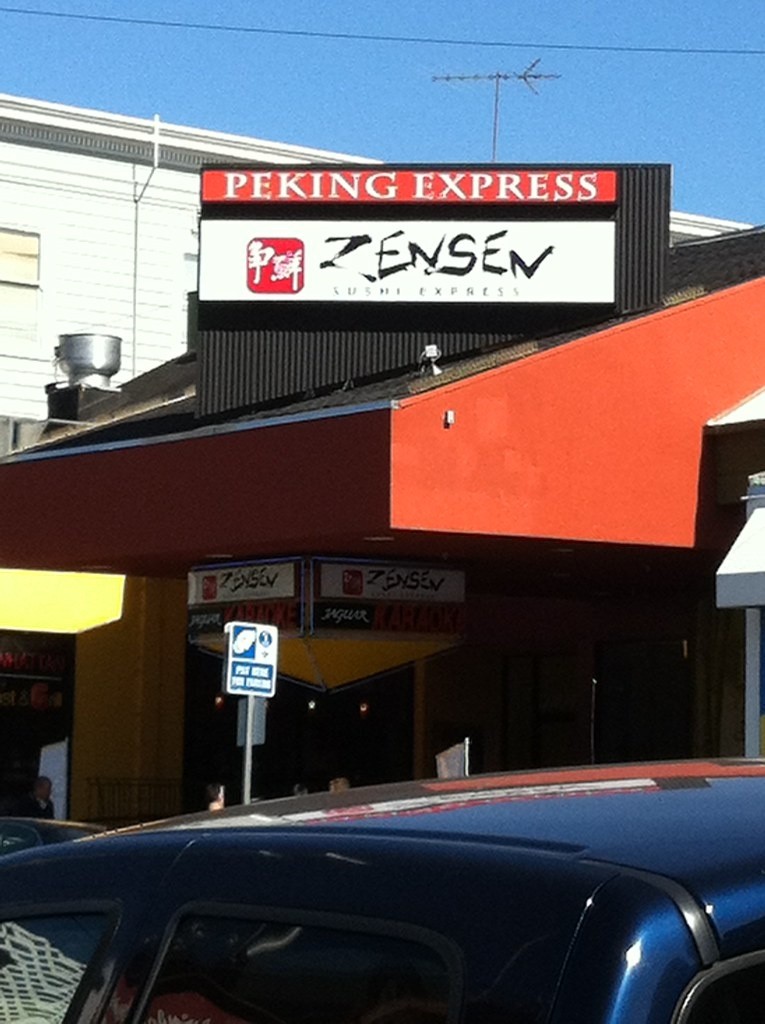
[13, 776, 55, 820]
